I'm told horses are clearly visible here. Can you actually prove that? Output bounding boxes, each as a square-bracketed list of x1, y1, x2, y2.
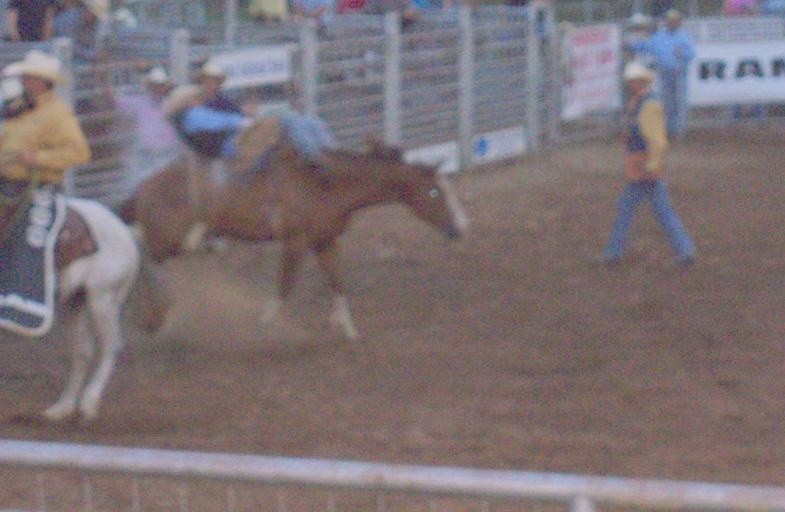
[0, 195, 147, 425]
[114, 133, 471, 359]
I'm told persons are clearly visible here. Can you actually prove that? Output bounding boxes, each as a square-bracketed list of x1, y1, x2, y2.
[580, 60, 700, 274]
[0, 47, 93, 268]
[169, 54, 370, 189]
[643, 6, 694, 140]
[0, 1, 549, 54]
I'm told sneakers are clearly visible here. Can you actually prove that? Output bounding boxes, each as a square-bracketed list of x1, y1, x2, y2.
[587, 252, 621, 273]
[667, 257, 698, 276]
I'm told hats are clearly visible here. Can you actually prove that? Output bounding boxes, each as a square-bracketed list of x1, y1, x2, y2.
[193, 62, 231, 83]
[139, 66, 178, 92]
[630, 8, 684, 28]
[2, 48, 74, 89]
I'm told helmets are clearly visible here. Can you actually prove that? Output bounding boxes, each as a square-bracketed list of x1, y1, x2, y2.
[620, 64, 654, 83]
